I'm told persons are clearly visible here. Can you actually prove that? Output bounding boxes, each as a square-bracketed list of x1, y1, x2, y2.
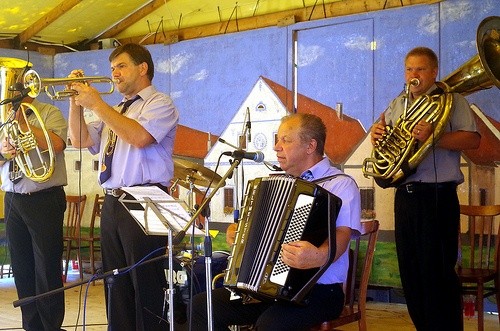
[67, 44, 179, 330]
[371, 48, 481, 331]
[193, 113, 367, 331]
[1, 71, 67, 331]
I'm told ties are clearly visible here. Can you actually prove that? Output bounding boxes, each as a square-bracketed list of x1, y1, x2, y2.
[301, 171, 313, 181]
[8, 109, 33, 184]
[100, 95, 141, 185]
[411, 142, 419, 176]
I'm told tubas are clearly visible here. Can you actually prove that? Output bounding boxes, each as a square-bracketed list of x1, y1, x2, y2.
[0, 55, 56, 183]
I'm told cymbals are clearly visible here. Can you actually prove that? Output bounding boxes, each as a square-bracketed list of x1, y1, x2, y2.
[172, 179, 203, 196]
[170, 158, 228, 189]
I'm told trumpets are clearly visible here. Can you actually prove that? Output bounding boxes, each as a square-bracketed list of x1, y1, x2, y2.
[23, 70, 120, 101]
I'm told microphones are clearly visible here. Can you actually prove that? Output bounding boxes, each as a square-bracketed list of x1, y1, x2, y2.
[224, 151, 265, 163]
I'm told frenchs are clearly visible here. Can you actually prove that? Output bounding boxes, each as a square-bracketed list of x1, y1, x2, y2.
[360, 16, 500, 189]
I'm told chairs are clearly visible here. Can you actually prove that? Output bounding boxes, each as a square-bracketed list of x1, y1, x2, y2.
[320, 220, 379, 331]
[63, 194, 104, 286]
[460, 204, 500, 331]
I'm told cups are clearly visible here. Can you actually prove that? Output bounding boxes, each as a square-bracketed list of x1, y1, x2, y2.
[463, 295, 476, 316]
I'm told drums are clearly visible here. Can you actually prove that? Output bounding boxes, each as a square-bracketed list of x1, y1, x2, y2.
[163, 250, 233, 309]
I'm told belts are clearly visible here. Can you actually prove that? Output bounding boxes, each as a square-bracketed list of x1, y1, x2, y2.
[106, 189, 130, 197]
[400, 183, 456, 193]
[15, 185, 62, 196]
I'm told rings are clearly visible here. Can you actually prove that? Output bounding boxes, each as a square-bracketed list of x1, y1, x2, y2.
[417, 130, 420, 134]
